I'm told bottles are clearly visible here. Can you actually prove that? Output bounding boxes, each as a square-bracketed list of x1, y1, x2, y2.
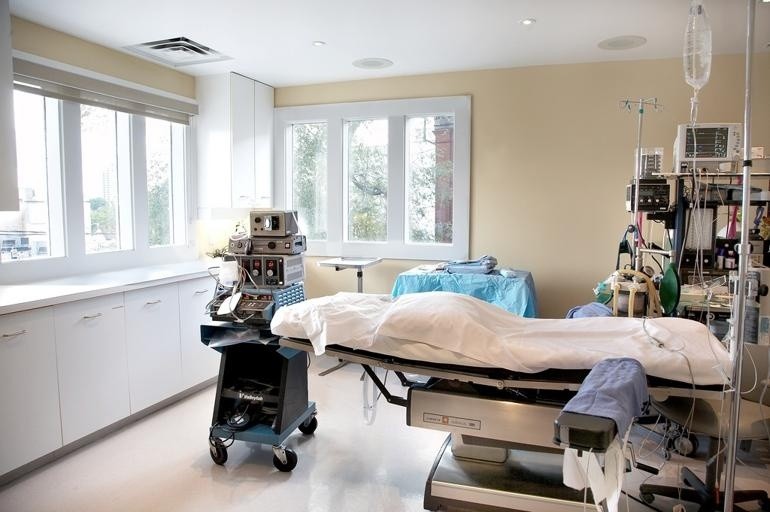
[725, 251, 736, 269]
[724, 243, 730, 258]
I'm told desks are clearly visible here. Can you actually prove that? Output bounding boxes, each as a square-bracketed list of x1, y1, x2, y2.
[399, 263, 532, 318]
[684, 293, 761, 320]
[319, 256, 382, 293]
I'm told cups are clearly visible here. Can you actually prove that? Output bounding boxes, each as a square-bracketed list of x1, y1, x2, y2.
[717, 256, 724, 270]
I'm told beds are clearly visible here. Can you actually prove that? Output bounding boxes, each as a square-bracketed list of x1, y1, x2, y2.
[269, 294, 725, 399]
